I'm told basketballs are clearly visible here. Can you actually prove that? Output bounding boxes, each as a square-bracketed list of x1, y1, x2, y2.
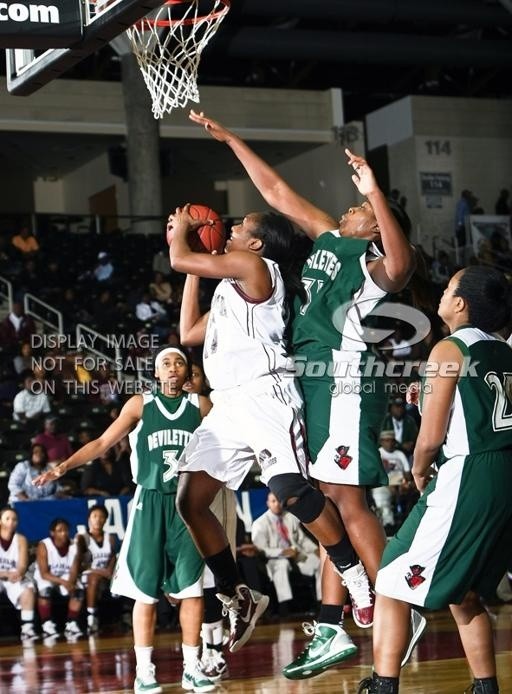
[166, 205, 225, 254]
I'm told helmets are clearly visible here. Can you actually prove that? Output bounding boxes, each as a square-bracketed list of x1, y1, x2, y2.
[388, 398, 407, 413]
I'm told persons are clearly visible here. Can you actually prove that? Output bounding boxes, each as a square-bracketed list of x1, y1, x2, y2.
[0, 108, 512, 694]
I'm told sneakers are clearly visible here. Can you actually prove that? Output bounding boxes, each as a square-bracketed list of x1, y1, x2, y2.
[20, 615, 99, 641]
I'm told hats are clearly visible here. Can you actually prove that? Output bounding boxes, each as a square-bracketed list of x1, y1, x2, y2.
[380, 430, 395, 439]
